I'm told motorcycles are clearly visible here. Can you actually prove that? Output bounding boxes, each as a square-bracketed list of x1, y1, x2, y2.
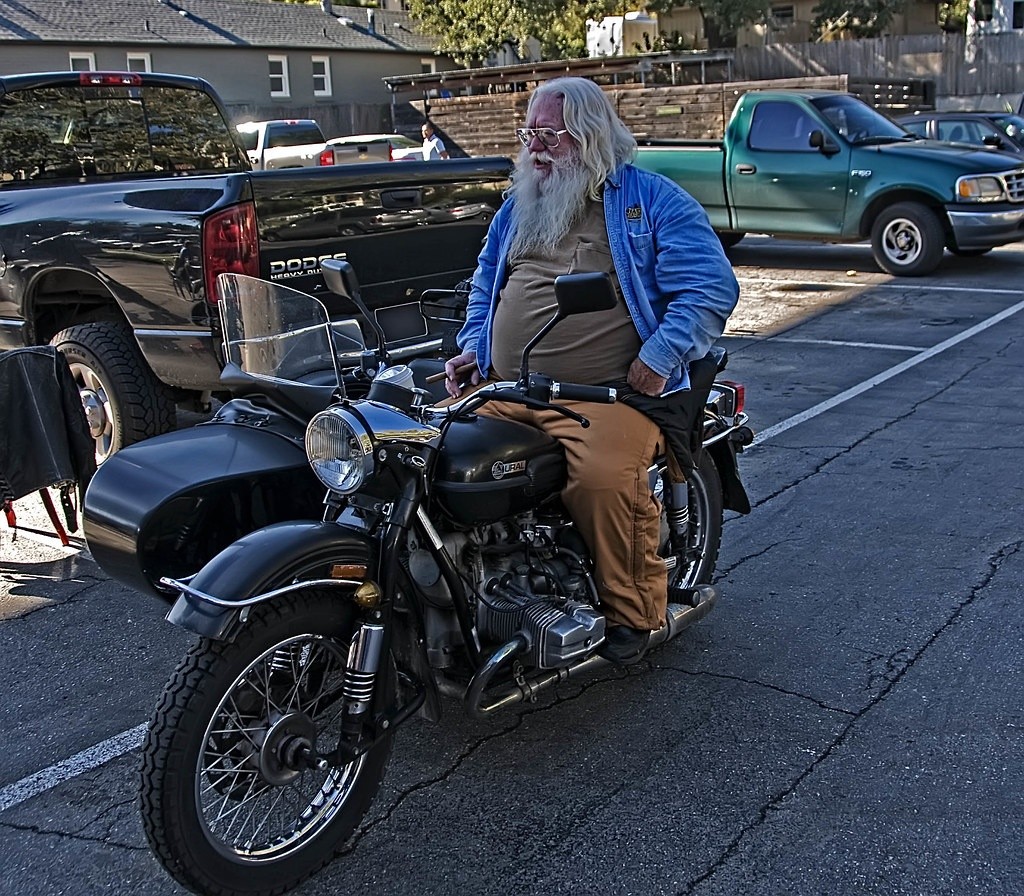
[77, 271, 756, 896]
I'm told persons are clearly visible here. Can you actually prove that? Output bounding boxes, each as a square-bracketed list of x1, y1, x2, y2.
[444, 79, 740, 665]
[421, 123, 447, 161]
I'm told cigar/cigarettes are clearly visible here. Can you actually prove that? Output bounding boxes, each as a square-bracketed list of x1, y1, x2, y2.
[426, 361, 479, 384]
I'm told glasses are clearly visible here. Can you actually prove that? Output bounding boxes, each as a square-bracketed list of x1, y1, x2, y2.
[514, 127, 568, 148]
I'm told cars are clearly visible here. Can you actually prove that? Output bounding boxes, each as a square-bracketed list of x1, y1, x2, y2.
[322, 135, 423, 168]
[887, 111, 1024, 153]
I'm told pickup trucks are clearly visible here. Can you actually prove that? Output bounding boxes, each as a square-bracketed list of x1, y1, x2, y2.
[622, 89, 1024, 276]
[0, 68, 514, 480]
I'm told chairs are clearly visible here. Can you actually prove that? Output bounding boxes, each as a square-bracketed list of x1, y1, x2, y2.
[794, 116, 811, 137]
[948, 126, 964, 141]
[838, 108, 858, 135]
[408, 357, 450, 403]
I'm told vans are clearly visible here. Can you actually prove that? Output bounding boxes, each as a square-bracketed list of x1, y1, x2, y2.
[232, 118, 334, 175]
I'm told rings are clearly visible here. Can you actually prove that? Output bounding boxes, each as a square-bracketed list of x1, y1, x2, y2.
[639, 386, 647, 392]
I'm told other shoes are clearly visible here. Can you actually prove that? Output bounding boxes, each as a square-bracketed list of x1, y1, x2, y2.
[595, 621, 650, 666]
